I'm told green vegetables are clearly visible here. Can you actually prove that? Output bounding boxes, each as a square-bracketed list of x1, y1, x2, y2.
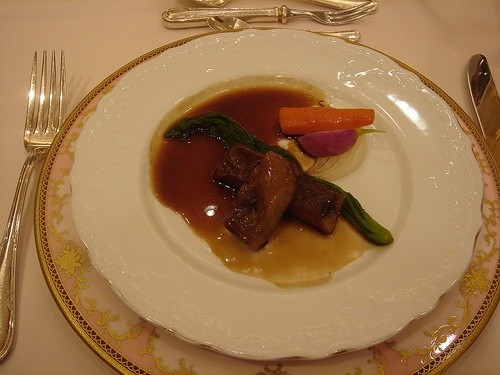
[164, 111, 394, 247]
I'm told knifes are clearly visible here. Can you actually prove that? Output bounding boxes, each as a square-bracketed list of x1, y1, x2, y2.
[467, 53, 500, 172]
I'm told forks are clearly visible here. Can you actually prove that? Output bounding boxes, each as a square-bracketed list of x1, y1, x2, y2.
[161, 2, 378, 30]
[0, 50, 68, 364]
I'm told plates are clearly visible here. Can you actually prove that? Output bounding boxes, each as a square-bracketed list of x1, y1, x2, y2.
[34, 27, 500, 374]
[70, 30, 487, 362]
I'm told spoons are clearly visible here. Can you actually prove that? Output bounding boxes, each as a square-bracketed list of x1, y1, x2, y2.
[195, 0, 378, 15]
[206, 15, 362, 42]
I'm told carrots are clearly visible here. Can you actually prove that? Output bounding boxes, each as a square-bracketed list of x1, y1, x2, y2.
[280, 107, 376, 135]
